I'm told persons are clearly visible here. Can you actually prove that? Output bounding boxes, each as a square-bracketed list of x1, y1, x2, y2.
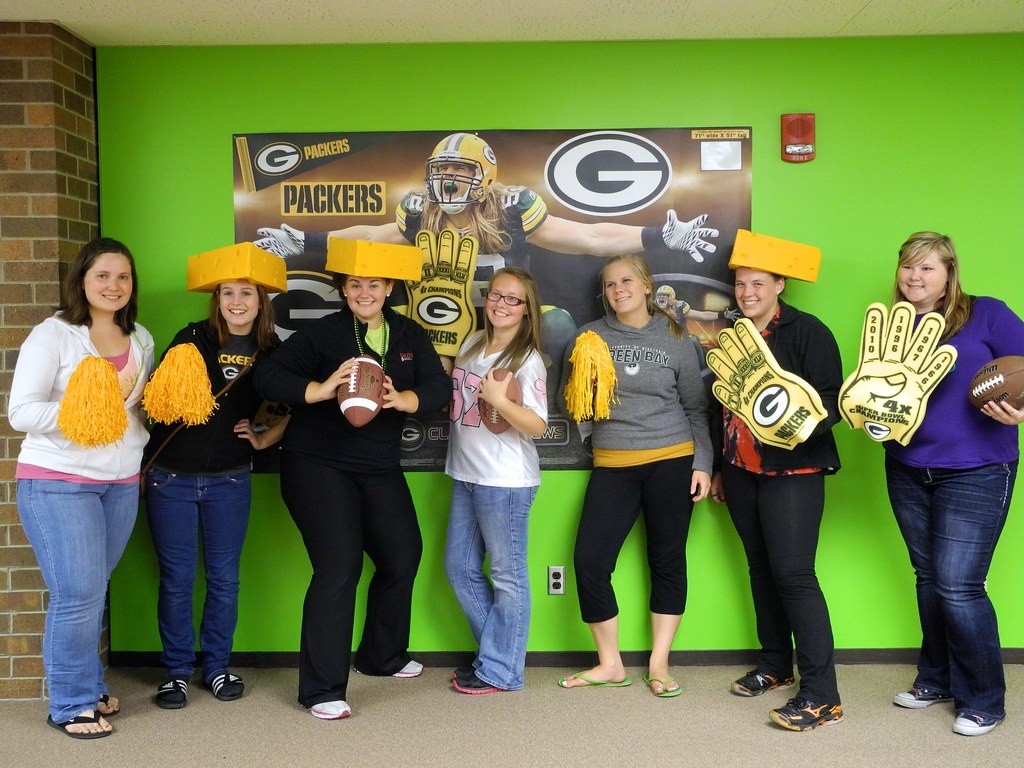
[145, 280, 292, 709]
[254, 133, 720, 414]
[557, 254, 714, 697]
[883, 230, 1024, 736]
[443, 267, 548, 694]
[7, 236, 155, 739]
[279, 275, 453, 720]
[708, 265, 843, 731]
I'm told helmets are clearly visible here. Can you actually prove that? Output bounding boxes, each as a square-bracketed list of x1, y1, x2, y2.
[426, 132, 499, 214]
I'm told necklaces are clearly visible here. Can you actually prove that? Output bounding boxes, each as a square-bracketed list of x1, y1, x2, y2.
[354, 310, 386, 371]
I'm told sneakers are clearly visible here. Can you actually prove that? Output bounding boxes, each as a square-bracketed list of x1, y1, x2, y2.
[769, 696, 844, 732]
[354, 660, 424, 679]
[732, 671, 797, 698]
[299, 696, 352, 721]
[894, 683, 955, 709]
[451, 662, 509, 695]
[951, 713, 1005, 737]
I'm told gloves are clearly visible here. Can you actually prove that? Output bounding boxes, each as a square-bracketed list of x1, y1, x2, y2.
[639, 208, 719, 264]
[840, 301, 958, 448]
[250, 223, 328, 259]
[404, 229, 478, 358]
[706, 315, 826, 451]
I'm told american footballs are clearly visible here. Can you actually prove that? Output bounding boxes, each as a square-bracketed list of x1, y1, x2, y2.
[336, 355, 387, 427]
[967, 355, 1024, 416]
[477, 368, 523, 436]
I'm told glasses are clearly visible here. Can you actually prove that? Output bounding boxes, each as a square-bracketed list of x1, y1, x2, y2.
[487, 291, 527, 308]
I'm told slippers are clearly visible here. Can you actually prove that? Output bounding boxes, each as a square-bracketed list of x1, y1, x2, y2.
[208, 670, 245, 701]
[156, 679, 189, 710]
[46, 712, 112, 739]
[557, 670, 632, 691]
[96, 693, 121, 716]
[642, 669, 681, 698]
